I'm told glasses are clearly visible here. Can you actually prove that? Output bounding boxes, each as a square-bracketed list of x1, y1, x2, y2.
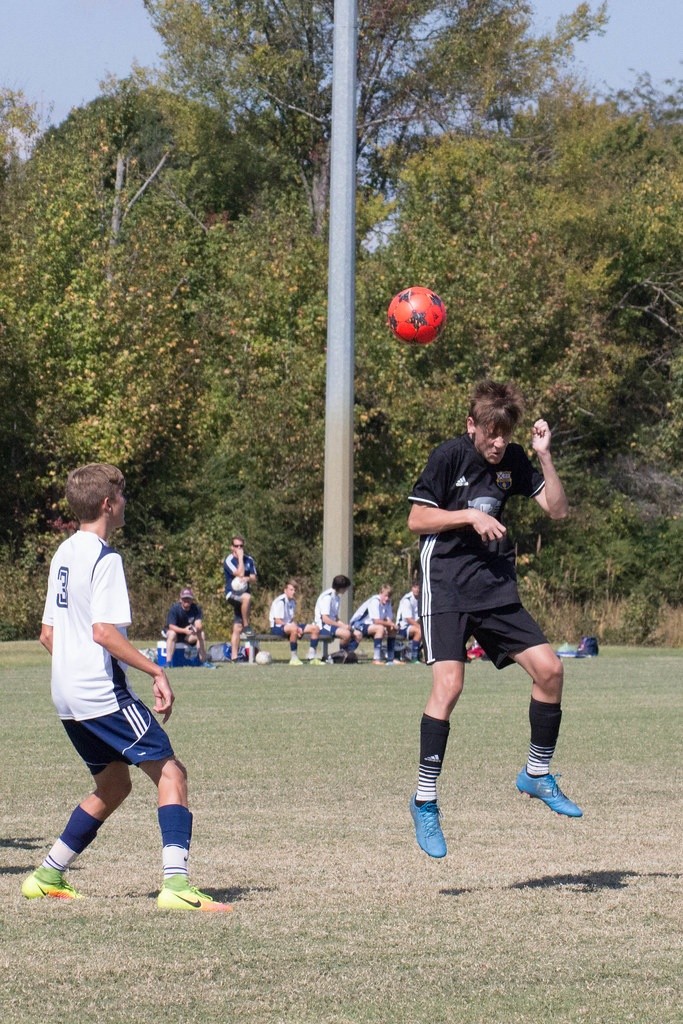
[232, 543, 243, 548]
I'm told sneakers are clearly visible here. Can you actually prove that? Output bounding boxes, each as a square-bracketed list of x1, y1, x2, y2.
[21, 874, 88, 899]
[371, 658, 387, 665]
[201, 661, 214, 668]
[385, 658, 405, 665]
[231, 658, 245, 664]
[309, 658, 326, 666]
[290, 658, 303, 665]
[244, 626, 255, 636]
[517, 764, 582, 817]
[410, 792, 447, 858]
[164, 662, 171, 669]
[158, 886, 234, 912]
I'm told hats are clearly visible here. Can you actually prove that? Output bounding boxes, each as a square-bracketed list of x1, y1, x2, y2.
[180, 588, 194, 599]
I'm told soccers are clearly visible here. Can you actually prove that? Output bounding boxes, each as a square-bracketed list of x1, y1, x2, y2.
[254, 651, 273, 666]
[386, 285, 447, 347]
[230, 576, 248, 593]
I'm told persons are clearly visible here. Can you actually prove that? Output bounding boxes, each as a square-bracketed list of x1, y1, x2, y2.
[314, 575, 363, 652]
[269, 579, 326, 666]
[223, 536, 257, 665]
[160, 588, 217, 669]
[22, 464, 236, 914]
[408, 381, 584, 857]
[350, 584, 407, 666]
[396, 578, 423, 664]
